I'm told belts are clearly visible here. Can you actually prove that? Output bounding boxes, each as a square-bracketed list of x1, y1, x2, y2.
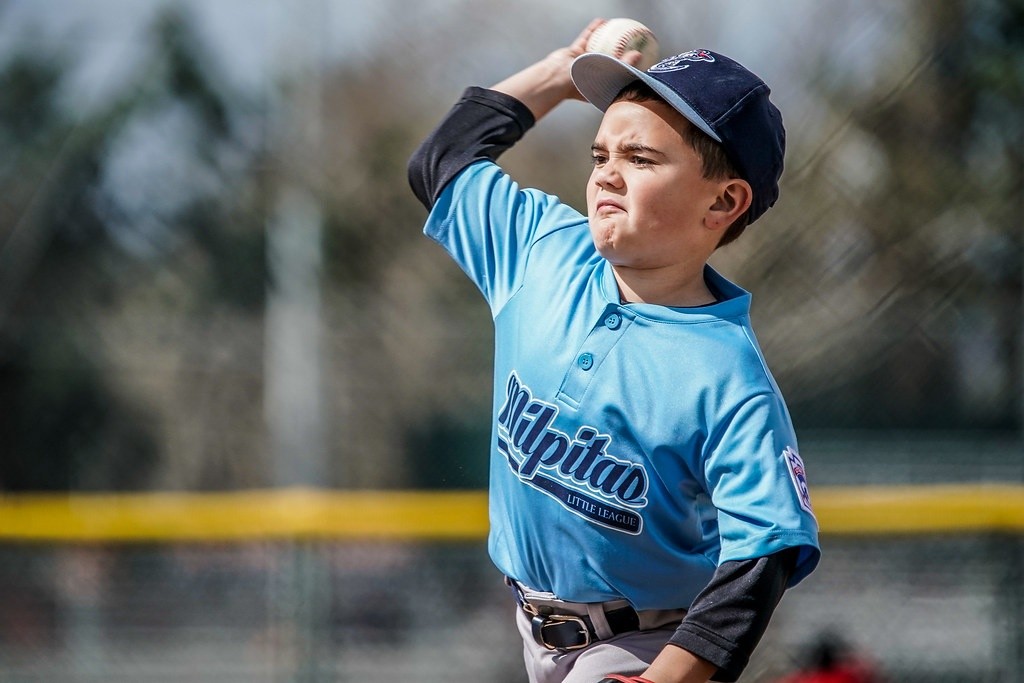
[510, 580, 639, 651]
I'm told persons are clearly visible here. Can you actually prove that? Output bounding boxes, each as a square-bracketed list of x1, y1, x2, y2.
[406, 18, 823, 683]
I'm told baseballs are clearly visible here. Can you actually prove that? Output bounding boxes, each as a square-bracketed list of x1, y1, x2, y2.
[585, 17, 662, 71]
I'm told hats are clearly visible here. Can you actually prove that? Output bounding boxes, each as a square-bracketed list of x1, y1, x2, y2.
[572, 49, 785, 225]
